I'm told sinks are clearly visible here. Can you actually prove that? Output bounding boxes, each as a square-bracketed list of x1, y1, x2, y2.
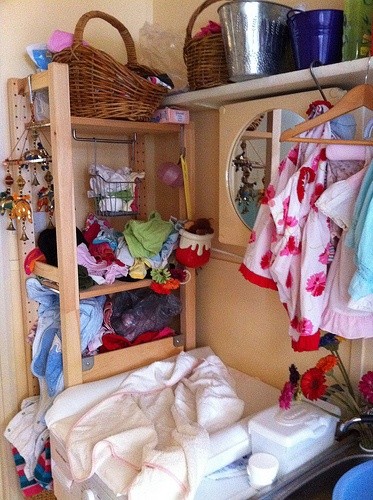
[332, 458, 373, 500]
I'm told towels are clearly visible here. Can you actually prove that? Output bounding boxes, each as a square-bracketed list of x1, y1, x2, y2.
[64, 347, 246, 500]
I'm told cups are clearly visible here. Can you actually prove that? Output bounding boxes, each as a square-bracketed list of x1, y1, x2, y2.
[247, 453, 280, 490]
[159, 161, 184, 189]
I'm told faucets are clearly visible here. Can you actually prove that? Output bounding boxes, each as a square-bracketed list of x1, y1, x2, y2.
[340, 414, 373, 432]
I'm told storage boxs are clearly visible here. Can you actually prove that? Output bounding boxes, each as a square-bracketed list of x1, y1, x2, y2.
[156, 106, 190, 124]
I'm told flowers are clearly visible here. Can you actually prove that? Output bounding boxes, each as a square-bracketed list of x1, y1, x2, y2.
[277, 327, 373, 452]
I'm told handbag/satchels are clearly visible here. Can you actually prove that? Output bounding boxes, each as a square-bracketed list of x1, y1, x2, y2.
[110, 290, 184, 345]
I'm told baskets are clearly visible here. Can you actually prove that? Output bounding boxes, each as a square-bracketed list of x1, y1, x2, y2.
[246, 113, 265, 131]
[89, 170, 141, 218]
[50, 8, 171, 122]
[183, 0, 231, 89]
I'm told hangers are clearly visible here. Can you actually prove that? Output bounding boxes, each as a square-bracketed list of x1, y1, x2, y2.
[279, 49, 373, 146]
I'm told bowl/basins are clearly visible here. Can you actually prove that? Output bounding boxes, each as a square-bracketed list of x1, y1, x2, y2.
[332, 459, 373, 500]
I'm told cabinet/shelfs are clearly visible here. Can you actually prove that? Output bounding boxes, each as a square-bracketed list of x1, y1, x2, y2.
[5, 58, 197, 500]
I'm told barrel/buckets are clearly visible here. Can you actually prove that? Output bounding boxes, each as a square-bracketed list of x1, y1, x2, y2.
[216, 0, 294, 83]
[286, 8, 344, 71]
